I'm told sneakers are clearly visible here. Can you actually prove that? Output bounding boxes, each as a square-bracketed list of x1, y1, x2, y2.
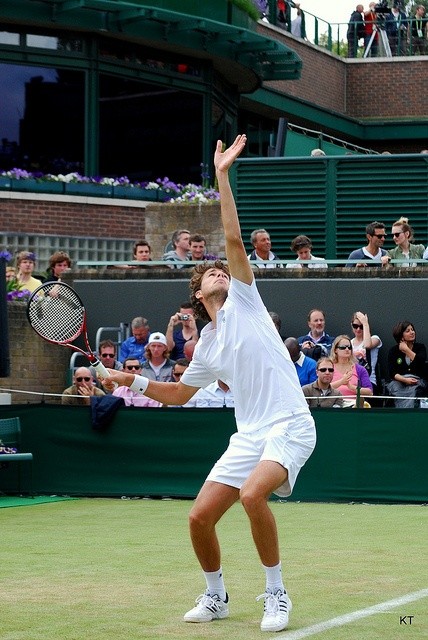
[183, 588, 229, 623]
[256, 586, 293, 632]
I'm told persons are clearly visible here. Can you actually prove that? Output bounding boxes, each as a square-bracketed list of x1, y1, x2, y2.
[188, 234, 218, 260]
[247, 228, 285, 269]
[204, 380, 234, 407]
[92, 131, 318, 633]
[160, 228, 191, 270]
[382, 215, 425, 269]
[301, 356, 343, 407]
[329, 333, 359, 406]
[42, 251, 73, 283]
[13, 250, 45, 299]
[387, 320, 427, 407]
[60, 366, 107, 406]
[133, 239, 152, 263]
[118, 316, 151, 365]
[88, 339, 123, 395]
[268, 311, 286, 342]
[139, 331, 177, 382]
[286, 236, 329, 269]
[111, 356, 163, 408]
[165, 301, 215, 360]
[345, 220, 390, 269]
[284, 335, 318, 390]
[349, 310, 383, 408]
[171, 360, 191, 382]
[253, 0, 428, 67]
[295, 308, 337, 356]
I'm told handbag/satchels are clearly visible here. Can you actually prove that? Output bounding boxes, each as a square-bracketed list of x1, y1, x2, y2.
[357, 347, 372, 377]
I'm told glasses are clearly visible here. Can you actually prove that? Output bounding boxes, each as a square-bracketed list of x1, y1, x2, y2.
[76, 376, 90, 382]
[172, 372, 183, 377]
[390, 232, 405, 237]
[125, 366, 140, 370]
[352, 321, 363, 330]
[335, 346, 352, 350]
[101, 353, 115, 358]
[373, 234, 387, 238]
[316, 368, 334, 372]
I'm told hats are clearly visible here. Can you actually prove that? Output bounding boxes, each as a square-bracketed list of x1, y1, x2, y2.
[144, 331, 167, 351]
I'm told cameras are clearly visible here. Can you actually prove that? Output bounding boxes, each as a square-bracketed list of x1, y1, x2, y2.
[178, 314, 189, 321]
